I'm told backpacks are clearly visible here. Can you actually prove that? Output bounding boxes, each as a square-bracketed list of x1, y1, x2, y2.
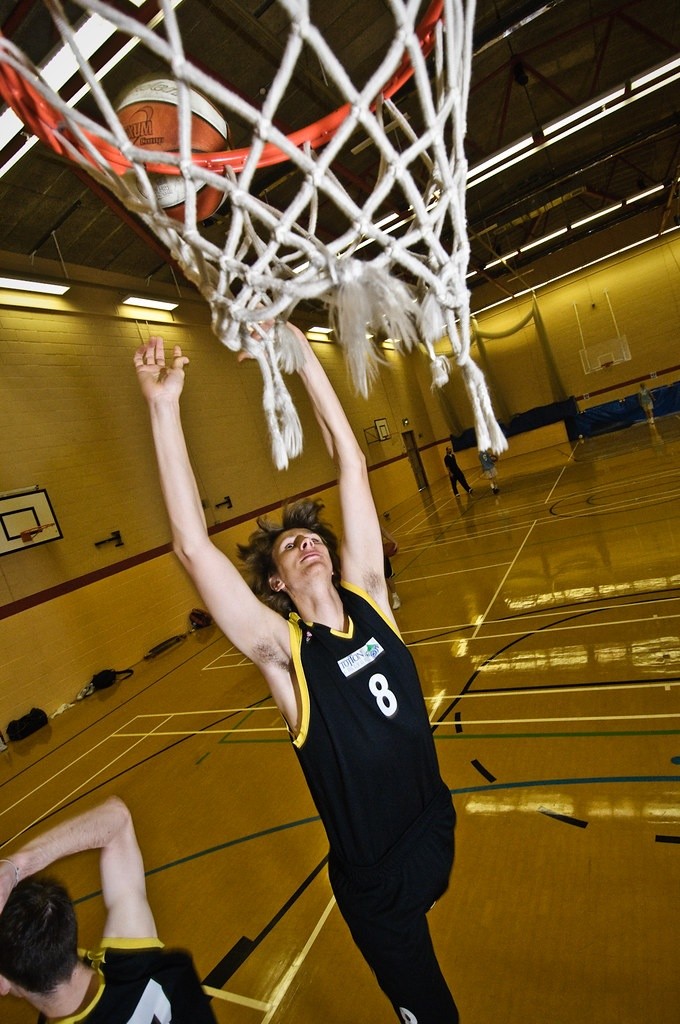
[189, 610, 212, 629]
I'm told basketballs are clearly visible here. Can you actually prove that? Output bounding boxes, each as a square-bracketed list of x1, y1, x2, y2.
[110, 78, 234, 223]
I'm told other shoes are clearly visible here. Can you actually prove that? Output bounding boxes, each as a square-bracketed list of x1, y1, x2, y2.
[0, 737, 8, 752]
[468, 489, 473, 494]
[492, 488, 499, 494]
[393, 596, 400, 609]
[456, 494, 461, 497]
[648, 417, 654, 424]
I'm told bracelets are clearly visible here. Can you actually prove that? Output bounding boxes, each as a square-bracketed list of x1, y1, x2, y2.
[0, 859, 19, 885]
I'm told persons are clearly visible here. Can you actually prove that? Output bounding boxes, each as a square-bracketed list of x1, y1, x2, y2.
[444, 446, 473, 497]
[638, 382, 656, 424]
[0, 792, 219, 1024]
[133, 291, 456, 1024]
[479, 449, 500, 495]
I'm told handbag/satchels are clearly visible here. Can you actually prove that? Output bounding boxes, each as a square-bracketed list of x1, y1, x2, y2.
[6, 707, 49, 743]
[83, 668, 134, 698]
